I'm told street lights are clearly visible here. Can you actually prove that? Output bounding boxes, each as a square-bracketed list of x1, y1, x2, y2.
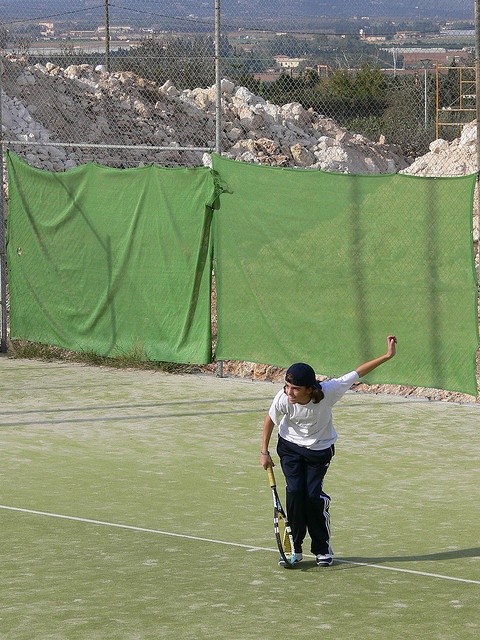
[420, 59, 431, 131]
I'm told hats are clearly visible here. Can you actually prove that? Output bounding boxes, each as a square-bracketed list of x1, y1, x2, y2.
[286, 363, 322, 390]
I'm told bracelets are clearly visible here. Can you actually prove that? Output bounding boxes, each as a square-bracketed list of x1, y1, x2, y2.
[261, 451, 270, 456]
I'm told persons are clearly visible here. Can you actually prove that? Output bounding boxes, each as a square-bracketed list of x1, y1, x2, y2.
[260, 335, 399, 566]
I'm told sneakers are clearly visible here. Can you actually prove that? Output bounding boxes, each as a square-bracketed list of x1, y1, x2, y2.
[279, 552, 303, 567]
[315, 554, 333, 566]
